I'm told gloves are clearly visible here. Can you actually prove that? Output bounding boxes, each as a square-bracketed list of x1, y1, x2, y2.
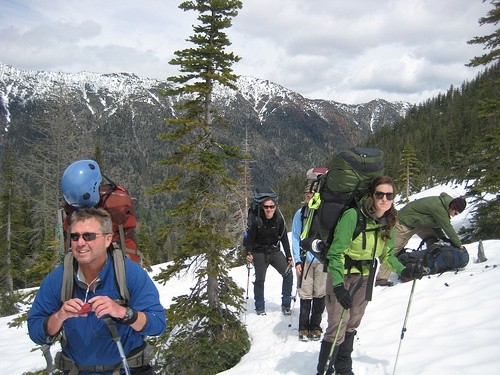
[333, 286, 354, 310]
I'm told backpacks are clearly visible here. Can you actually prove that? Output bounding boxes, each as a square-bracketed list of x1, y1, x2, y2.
[63, 184, 138, 263]
[299, 147, 385, 272]
[397, 236, 469, 283]
[246, 187, 278, 229]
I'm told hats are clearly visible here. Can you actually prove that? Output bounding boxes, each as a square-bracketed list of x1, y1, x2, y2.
[449, 198, 466, 213]
[304, 184, 317, 194]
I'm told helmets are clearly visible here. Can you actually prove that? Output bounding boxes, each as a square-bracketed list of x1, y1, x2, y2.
[61, 160, 103, 207]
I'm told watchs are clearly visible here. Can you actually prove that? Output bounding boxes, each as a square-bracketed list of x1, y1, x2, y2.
[120, 308, 133, 323]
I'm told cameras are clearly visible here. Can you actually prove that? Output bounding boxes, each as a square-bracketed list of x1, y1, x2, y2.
[76, 302, 92, 314]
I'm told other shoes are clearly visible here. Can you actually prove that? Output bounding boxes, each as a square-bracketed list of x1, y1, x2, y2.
[298, 329, 309, 342]
[256, 310, 267, 317]
[374, 280, 394, 288]
[282, 306, 291, 316]
[310, 329, 322, 341]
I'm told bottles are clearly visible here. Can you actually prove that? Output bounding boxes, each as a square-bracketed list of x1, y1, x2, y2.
[244, 229, 248, 238]
[311, 239, 325, 253]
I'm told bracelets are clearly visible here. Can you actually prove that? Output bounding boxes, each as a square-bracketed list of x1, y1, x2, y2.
[125, 309, 138, 325]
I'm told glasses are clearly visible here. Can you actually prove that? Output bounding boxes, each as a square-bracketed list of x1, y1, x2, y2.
[454, 209, 458, 215]
[374, 191, 395, 200]
[263, 205, 276, 210]
[70, 232, 112, 241]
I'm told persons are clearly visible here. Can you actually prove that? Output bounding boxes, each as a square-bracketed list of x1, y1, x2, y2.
[316, 175, 423, 375]
[246, 197, 294, 315]
[375, 191, 466, 287]
[292, 184, 328, 341]
[27, 206, 167, 375]
